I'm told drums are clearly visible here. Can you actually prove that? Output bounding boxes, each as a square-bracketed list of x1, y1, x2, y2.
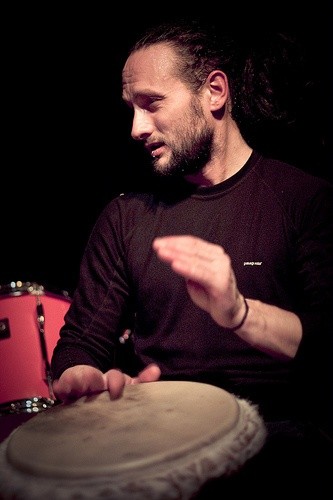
[0, 381, 268, 500]
[0, 281, 73, 414]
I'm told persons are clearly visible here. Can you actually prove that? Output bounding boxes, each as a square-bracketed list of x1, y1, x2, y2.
[50, 25, 333, 398]
[63, 290, 68, 297]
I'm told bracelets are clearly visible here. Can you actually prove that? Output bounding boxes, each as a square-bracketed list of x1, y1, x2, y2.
[228, 296, 249, 331]
